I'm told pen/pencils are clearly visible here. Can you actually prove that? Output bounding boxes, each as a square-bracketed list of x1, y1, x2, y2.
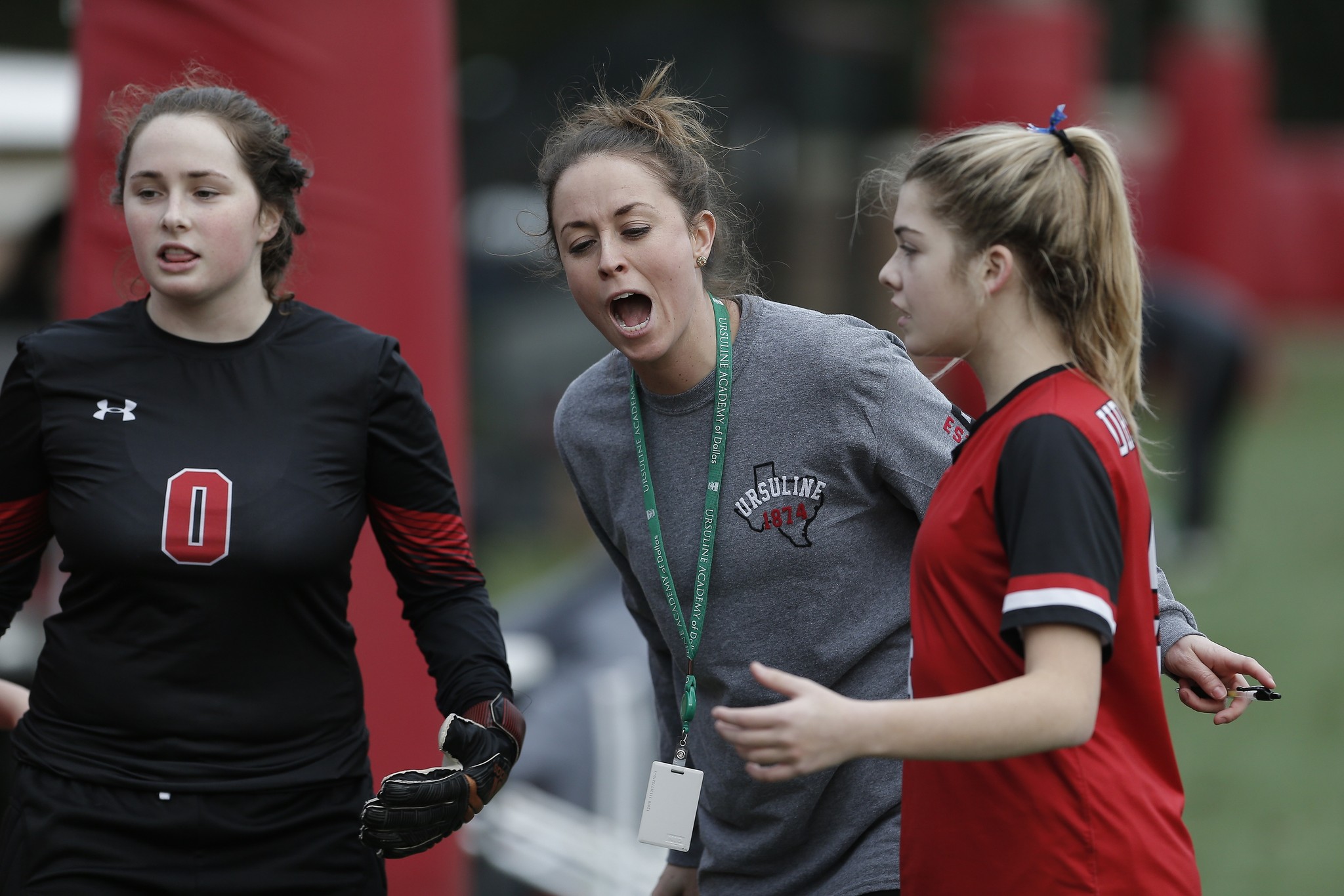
[1174, 686, 1282, 702]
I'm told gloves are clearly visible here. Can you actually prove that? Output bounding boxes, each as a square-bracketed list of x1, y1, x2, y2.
[356, 690, 530, 862]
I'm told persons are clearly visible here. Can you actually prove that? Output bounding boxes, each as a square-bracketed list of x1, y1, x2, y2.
[708, 98, 1206, 895]
[0, 58, 528, 895]
[514, 48, 1277, 896]
